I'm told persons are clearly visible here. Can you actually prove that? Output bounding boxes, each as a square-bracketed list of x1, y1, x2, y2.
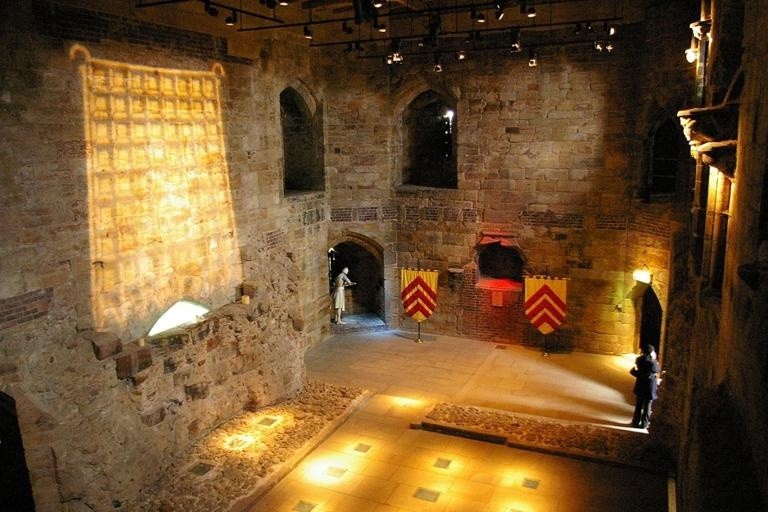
[633, 344, 660, 428]
[332, 267, 352, 324]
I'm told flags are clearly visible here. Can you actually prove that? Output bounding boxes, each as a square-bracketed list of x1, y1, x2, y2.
[524, 275, 567, 335]
[401, 267, 438, 323]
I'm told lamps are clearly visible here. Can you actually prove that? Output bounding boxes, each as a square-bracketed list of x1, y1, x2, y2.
[225, 2, 619, 74]
